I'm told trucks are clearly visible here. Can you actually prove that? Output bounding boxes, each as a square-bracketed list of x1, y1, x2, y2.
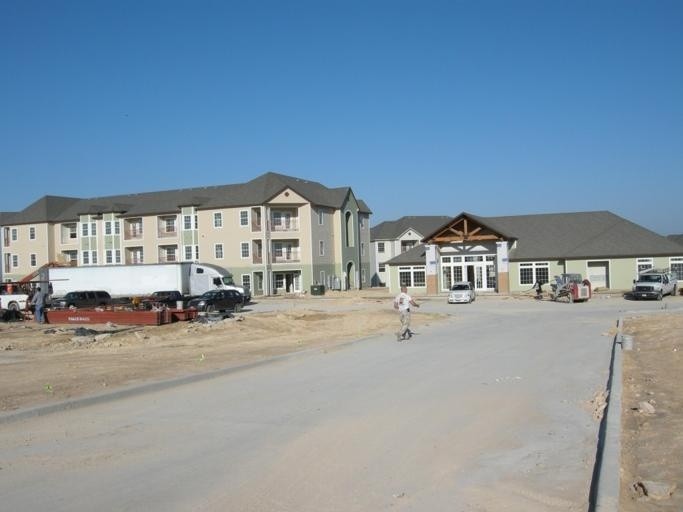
[632, 271, 678, 301]
[47, 262, 251, 308]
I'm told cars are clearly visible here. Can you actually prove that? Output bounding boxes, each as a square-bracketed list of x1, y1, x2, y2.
[448, 282, 476, 303]
[140, 289, 246, 313]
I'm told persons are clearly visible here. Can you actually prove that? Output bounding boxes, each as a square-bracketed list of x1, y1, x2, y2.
[533, 280, 543, 296]
[393, 286, 419, 339]
[31, 287, 46, 323]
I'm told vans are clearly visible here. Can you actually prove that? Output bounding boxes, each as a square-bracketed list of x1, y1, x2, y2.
[50, 291, 113, 310]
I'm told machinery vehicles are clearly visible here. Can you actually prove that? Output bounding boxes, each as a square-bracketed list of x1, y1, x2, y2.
[0, 262, 65, 312]
